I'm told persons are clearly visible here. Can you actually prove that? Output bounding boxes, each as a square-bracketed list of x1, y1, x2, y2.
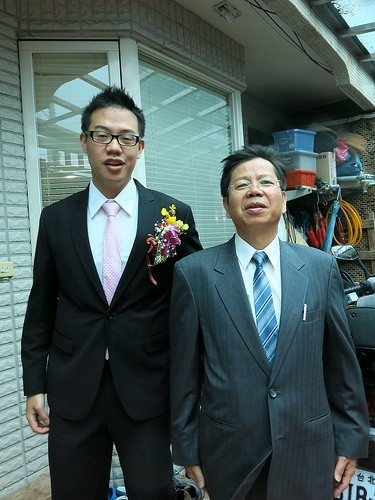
[22, 85, 203, 500]
[167, 144, 368, 500]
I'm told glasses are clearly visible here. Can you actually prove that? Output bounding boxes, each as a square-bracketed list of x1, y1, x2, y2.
[84, 129, 142, 146]
[227, 179, 280, 194]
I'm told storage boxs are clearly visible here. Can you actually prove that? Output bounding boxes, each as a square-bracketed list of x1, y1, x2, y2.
[285, 169, 315, 189]
[272, 129, 317, 152]
[315, 151, 337, 187]
[277, 151, 318, 172]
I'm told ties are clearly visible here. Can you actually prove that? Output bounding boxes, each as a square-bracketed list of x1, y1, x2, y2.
[252, 250, 280, 370]
[101, 199, 122, 306]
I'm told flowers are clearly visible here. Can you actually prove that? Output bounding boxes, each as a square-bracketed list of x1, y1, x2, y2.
[152, 204, 189, 266]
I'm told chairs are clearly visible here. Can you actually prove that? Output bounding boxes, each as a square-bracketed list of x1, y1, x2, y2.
[346, 307, 375, 426]
